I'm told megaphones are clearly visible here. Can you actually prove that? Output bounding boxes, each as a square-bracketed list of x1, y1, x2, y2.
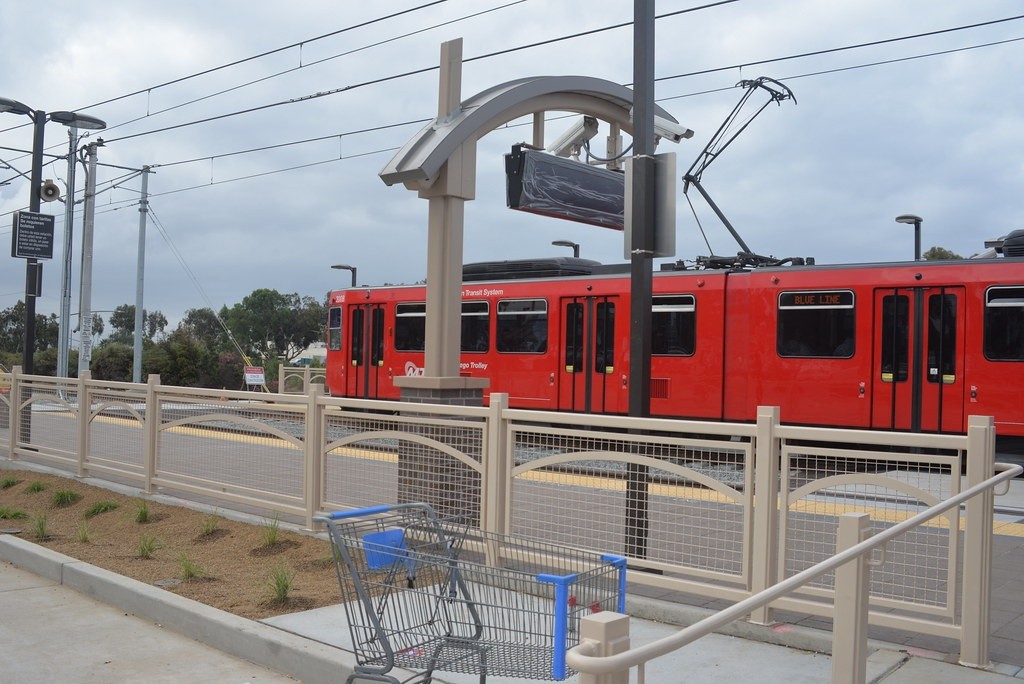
[41, 179, 59, 202]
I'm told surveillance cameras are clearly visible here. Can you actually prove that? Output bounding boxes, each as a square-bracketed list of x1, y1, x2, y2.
[628, 107, 693, 144]
[547, 116, 599, 159]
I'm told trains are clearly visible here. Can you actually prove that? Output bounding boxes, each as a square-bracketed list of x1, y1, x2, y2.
[326, 73, 1023, 461]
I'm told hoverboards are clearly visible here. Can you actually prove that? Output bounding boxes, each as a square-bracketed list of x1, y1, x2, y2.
[311, 500, 626, 684]
